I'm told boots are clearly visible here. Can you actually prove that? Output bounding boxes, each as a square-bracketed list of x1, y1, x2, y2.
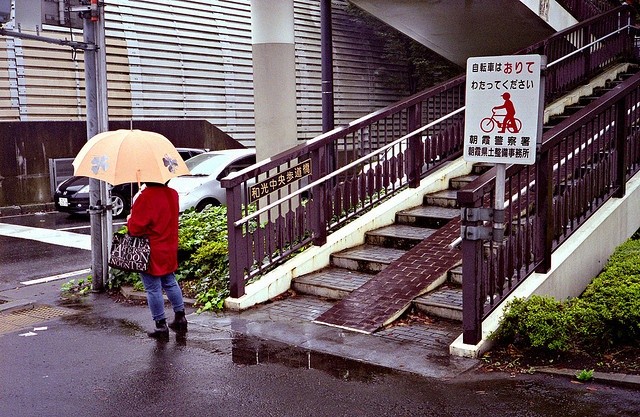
[168, 309, 188, 328]
[148, 319, 170, 338]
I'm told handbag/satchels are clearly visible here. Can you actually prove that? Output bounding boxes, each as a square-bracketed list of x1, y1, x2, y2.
[108, 230, 151, 274]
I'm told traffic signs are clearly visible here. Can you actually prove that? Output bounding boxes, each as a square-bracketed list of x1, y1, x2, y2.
[463, 53, 542, 166]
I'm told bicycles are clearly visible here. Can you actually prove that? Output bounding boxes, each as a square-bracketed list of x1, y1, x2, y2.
[479, 107, 523, 137]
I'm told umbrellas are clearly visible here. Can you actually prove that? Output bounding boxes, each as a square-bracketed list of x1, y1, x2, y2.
[71, 119, 192, 215]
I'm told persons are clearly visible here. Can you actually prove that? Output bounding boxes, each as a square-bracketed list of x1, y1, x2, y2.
[126, 179, 188, 338]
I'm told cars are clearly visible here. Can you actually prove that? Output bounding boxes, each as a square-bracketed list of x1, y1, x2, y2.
[56, 145, 209, 218]
[133, 149, 260, 221]
